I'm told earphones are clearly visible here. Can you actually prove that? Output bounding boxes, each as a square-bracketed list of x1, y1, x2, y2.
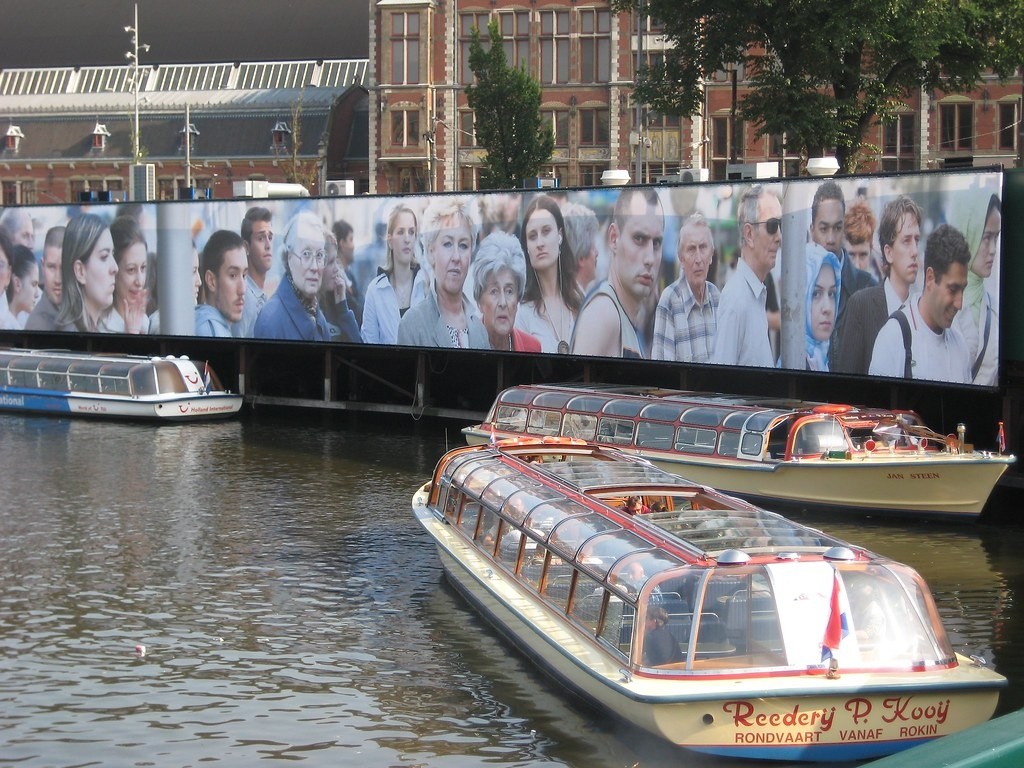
[558, 234, 562, 244]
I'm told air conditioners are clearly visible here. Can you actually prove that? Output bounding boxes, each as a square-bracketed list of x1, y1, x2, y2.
[680, 168, 711, 182]
[324, 179, 355, 198]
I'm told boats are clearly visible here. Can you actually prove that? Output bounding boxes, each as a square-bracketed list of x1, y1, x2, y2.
[0, 347, 243, 423]
[413, 436, 1008, 762]
[461, 383, 1017, 524]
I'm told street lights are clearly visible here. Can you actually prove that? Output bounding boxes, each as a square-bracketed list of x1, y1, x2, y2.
[124, 4, 150, 162]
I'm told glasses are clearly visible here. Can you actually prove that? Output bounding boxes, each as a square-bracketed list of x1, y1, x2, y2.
[744, 218, 783, 234]
[291, 250, 327, 265]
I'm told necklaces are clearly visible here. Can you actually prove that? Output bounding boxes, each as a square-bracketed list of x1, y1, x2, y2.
[490, 333, 512, 351]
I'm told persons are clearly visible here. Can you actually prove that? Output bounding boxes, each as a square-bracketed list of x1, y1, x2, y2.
[445, 455, 887, 665]
[0, 181, 1001, 385]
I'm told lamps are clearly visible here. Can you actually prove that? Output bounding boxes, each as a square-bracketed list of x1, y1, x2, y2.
[92, 122, 110, 148]
[5, 122, 24, 153]
[270, 120, 292, 150]
[178, 123, 201, 150]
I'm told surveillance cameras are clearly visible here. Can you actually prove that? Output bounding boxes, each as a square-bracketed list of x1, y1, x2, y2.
[645, 139, 652, 148]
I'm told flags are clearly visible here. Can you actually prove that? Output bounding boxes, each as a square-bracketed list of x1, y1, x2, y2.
[821, 576, 850, 662]
[997, 427, 1006, 452]
[204, 363, 211, 395]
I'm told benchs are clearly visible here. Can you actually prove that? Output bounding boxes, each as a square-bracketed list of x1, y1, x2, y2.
[458, 498, 913, 666]
[528, 422, 820, 459]
